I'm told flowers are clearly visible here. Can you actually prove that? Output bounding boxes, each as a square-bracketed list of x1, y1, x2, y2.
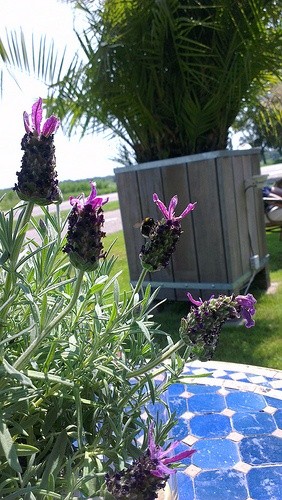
[0, 96, 260, 500]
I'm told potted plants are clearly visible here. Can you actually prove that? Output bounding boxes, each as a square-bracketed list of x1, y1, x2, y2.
[0, 0, 282, 320]
